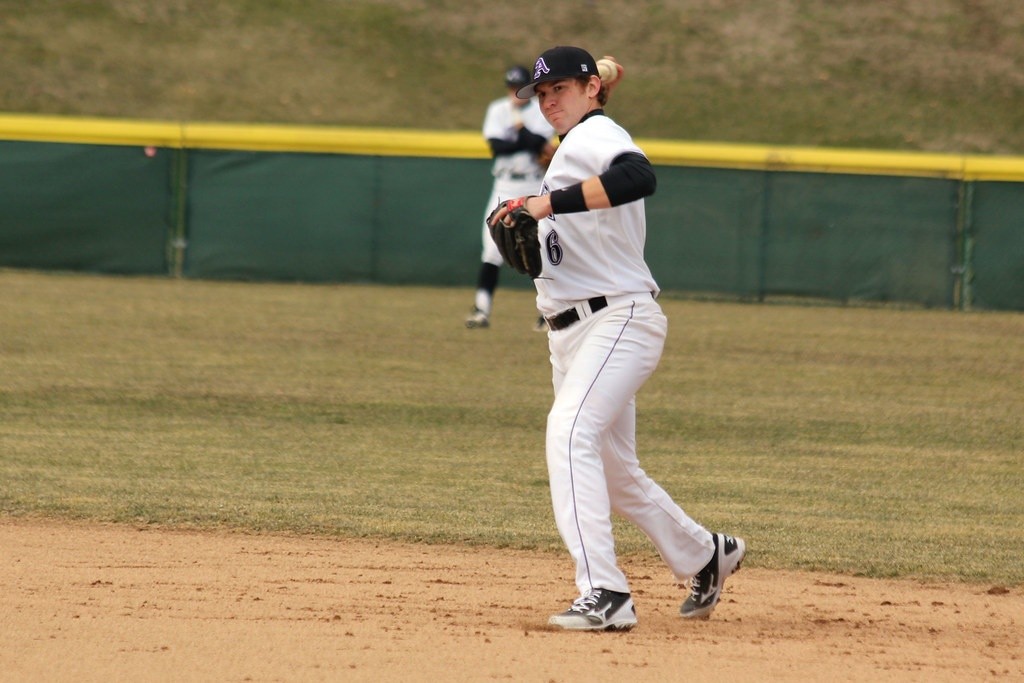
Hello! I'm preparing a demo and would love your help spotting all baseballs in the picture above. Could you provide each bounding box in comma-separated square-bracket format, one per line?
[594, 58, 619, 85]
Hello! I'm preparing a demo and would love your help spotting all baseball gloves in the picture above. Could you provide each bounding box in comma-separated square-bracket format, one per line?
[484, 194, 546, 280]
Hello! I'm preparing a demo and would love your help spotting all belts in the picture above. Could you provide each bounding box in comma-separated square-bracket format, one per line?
[510, 172, 540, 180]
[545, 296, 608, 331]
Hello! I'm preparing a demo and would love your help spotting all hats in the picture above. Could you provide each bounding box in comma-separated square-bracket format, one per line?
[505, 66, 531, 86]
[515, 45, 600, 99]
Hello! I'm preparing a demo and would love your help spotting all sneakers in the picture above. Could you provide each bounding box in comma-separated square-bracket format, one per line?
[547, 588, 638, 632]
[677, 532, 747, 618]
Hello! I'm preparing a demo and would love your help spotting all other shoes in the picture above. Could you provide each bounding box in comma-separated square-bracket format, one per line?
[465, 304, 489, 328]
[532, 309, 550, 332]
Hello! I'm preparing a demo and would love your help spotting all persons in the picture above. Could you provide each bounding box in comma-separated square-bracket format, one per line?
[464, 64, 554, 333]
[490, 45, 746, 633]
